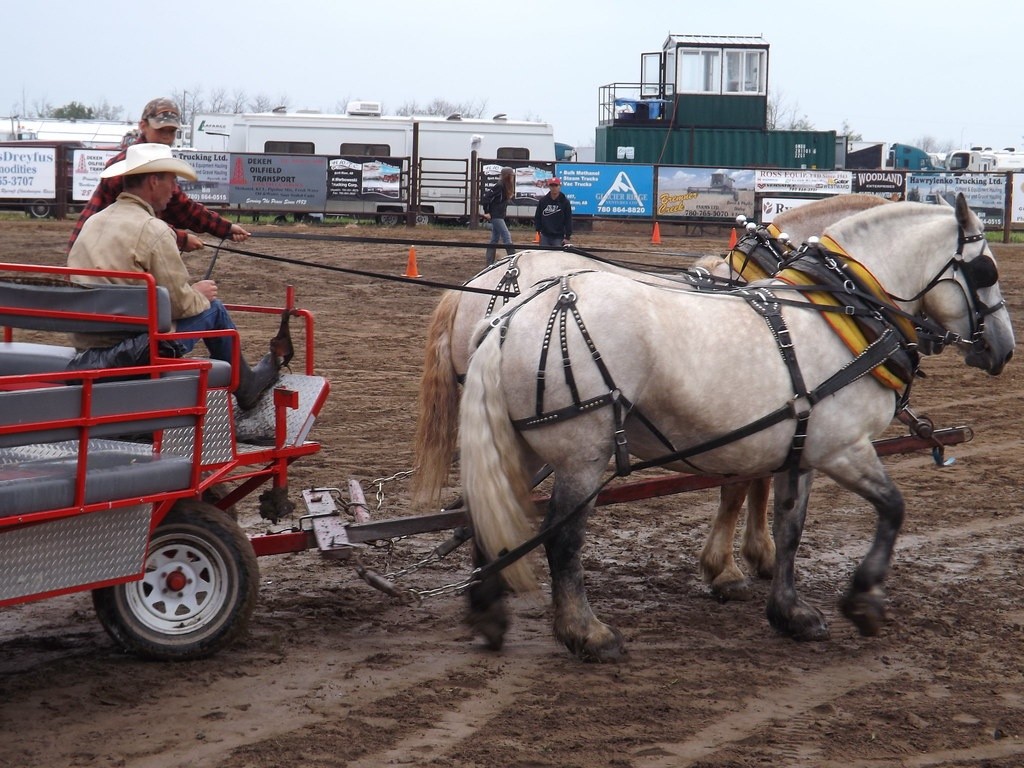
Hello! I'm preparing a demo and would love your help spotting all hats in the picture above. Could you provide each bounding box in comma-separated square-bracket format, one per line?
[100, 143, 197, 181]
[548, 177, 560, 185]
[141, 97, 184, 131]
[501, 167, 515, 176]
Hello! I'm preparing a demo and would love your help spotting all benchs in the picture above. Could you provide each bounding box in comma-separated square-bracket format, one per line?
[0, 357, 212, 528]
[0, 262, 241, 391]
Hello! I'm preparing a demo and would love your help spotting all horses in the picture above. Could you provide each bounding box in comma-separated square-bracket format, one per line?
[412, 184, 902, 603]
[455, 190, 1017, 661]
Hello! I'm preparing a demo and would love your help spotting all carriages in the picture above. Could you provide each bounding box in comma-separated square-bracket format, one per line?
[0, 192, 1016, 666]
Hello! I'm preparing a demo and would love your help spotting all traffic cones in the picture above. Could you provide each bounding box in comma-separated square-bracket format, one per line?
[650, 221, 662, 244]
[725, 227, 737, 251]
[401, 245, 422, 278]
[532, 231, 541, 243]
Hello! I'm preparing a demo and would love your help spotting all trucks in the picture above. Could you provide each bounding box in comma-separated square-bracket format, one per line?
[944, 147, 1024, 177]
[0, 116, 192, 219]
[190, 102, 578, 227]
[847, 141, 935, 176]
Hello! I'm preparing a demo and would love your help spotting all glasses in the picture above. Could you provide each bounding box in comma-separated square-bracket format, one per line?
[549, 183, 559, 187]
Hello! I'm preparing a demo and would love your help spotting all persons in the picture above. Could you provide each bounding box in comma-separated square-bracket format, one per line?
[66, 98, 253, 263]
[483, 166, 517, 268]
[67, 141, 281, 411]
[535, 177, 573, 247]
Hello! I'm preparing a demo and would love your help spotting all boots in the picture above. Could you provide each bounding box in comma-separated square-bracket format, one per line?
[208, 336, 280, 410]
[485, 248, 496, 266]
[506, 248, 515, 255]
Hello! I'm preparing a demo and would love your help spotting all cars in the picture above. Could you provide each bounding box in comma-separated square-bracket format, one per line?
[920, 194, 938, 203]
